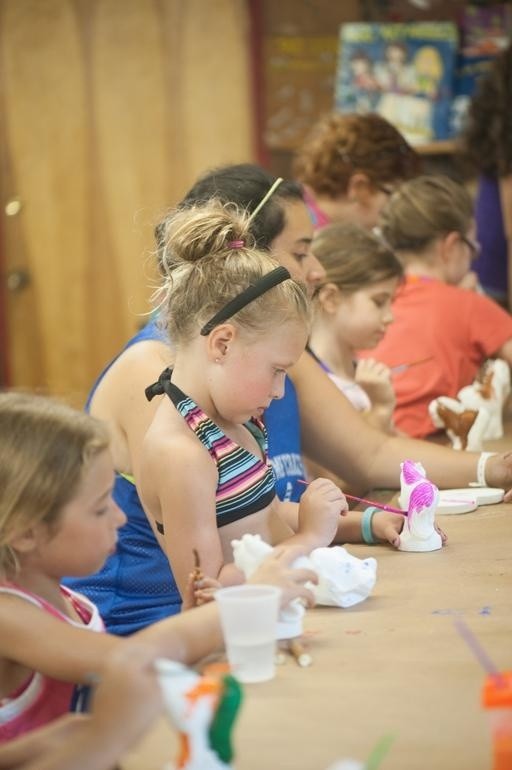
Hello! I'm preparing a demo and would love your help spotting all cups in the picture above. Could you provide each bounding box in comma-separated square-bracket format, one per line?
[217, 584, 280, 684]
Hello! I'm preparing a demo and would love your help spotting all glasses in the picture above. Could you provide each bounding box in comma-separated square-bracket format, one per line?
[463, 231, 484, 261]
[375, 182, 393, 197]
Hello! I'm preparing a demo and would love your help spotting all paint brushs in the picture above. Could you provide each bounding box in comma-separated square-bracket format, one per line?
[297, 478, 408, 516]
[193, 549, 202, 605]
[390, 355, 436, 372]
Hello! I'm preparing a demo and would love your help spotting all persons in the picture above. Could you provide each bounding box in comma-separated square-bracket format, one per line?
[0, 390, 320, 769]
[336, 22, 456, 140]
[56, 165, 512, 564]
[140, 203, 449, 597]
[358, 174, 512, 437]
[294, 113, 437, 223]
[297, 217, 438, 439]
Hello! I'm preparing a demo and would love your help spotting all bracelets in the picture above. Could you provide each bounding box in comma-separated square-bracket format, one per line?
[469, 452, 497, 488]
[361, 506, 378, 543]
